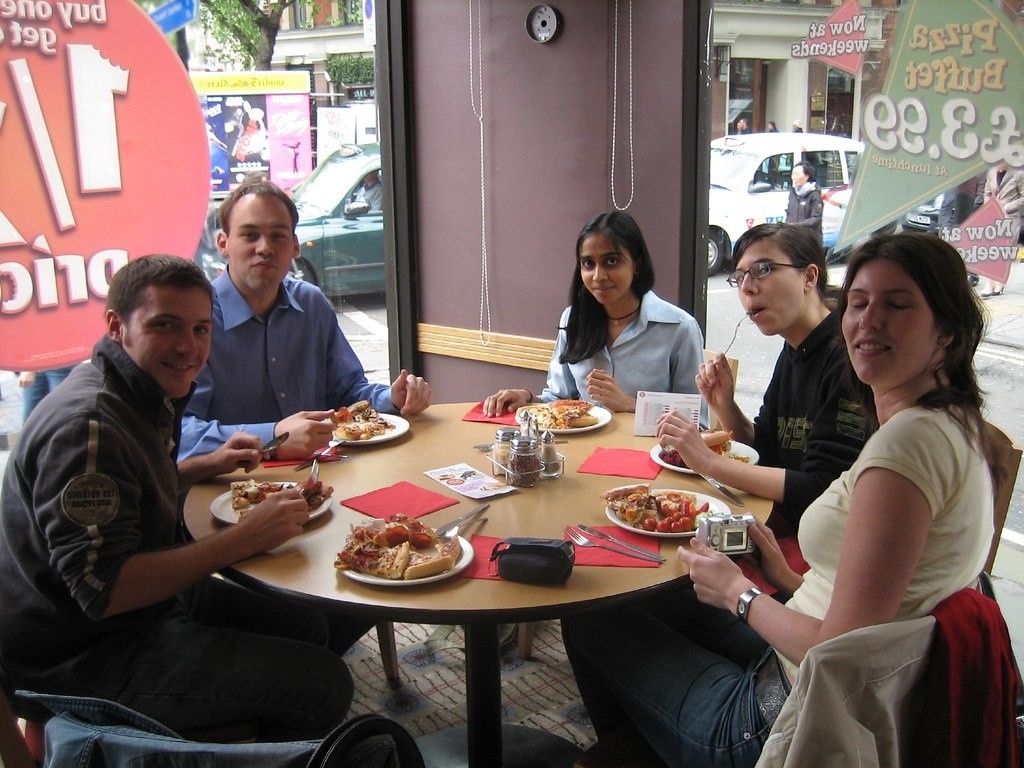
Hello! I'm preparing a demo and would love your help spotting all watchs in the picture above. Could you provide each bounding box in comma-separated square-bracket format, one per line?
[736, 587, 764, 624]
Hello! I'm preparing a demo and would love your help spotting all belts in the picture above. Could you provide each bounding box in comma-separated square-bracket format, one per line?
[753, 652, 789, 725]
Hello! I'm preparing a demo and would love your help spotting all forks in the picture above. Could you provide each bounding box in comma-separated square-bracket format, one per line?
[292, 440, 347, 472]
[563, 524, 663, 562]
[723, 313, 750, 355]
[299, 458, 320, 495]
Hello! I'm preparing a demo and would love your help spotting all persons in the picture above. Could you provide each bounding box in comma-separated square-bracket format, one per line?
[768, 121, 779, 133]
[937, 158, 1024, 300]
[655, 221, 881, 542]
[1, 251, 358, 768]
[736, 119, 752, 135]
[173, 180, 434, 460]
[791, 120, 803, 133]
[785, 160, 824, 248]
[561, 231, 999, 768]
[482, 210, 710, 431]
[354, 170, 383, 211]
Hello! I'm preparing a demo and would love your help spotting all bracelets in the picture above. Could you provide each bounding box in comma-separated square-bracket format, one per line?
[522, 388, 534, 403]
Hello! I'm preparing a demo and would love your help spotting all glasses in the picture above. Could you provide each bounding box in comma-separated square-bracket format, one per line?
[726, 262, 802, 288]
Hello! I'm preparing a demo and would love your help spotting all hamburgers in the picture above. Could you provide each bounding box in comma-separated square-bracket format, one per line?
[699, 428, 732, 455]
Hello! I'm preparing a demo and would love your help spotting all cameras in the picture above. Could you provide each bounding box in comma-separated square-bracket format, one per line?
[694, 513, 756, 554]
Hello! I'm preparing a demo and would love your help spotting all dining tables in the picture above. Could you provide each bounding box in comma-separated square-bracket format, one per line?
[183, 402, 776, 767]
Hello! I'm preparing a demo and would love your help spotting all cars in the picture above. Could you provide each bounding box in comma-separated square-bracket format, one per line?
[210, 143, 386, 300]
[708, 132, 868, 277]
[901, 192, 949, 235]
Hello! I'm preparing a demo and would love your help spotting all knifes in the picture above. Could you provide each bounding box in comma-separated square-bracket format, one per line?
[700, 474, 745, 507]
[236, 432, 290, 465]
[576, 525, 666, 561]
[434, 504, 489, 536]
[313, 451, 348, 458]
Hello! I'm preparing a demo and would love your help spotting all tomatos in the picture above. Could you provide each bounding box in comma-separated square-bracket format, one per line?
[644, 512, 693, 533]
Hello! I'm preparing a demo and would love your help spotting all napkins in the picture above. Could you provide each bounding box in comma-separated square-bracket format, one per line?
[340, 482, 459, 522]
[259, 436, 341, 466]
[462, 396, 520, 426]
[579, 448, 662, 481]
[460, 530, 512, 582]
[565, 521, 665, 568]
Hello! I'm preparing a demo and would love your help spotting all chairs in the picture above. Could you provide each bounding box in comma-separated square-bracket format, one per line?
[743, 578, 985, 766]
[43, 695, 426, 767]
[966, 415, 1023, 576]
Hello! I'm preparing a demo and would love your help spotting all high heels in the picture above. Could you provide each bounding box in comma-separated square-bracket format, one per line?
[981, 287, 1004, 297]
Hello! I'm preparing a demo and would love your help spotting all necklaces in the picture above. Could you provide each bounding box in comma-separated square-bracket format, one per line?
[603, 298, 640, 326]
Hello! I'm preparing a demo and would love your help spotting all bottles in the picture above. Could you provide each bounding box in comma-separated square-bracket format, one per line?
[493, 409, 560, 486]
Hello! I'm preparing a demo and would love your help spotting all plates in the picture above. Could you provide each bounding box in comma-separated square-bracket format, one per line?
[210, 481, 332, 525]
[649, 433, 760, 474]
[319, 412, 411, 447]
[514, 405, 612, 435]
[606, 488, 731, 537]
[337, 528, 476, 586]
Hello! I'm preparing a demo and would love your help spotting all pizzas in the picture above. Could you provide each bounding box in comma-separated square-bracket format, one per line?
[231, 478, 333, 521]
[601, 484, 696, 526]
[516, 400, 598, 429]
[333, 513, 460, 580]
[331, 400, 396, 440]
